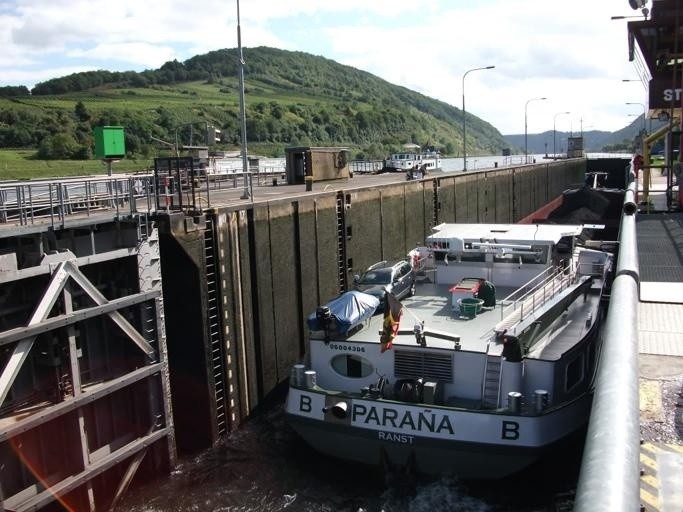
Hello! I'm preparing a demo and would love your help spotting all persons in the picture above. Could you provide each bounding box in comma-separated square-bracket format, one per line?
[419, 163, 427, 178]
[430, 242, 439, 249]
[406, 167, 415, 181]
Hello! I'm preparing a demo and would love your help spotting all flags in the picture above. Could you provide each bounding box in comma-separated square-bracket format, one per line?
[378, 292, 405, 354]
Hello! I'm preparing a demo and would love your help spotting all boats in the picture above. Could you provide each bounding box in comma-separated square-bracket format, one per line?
[385, 142, 441, 172]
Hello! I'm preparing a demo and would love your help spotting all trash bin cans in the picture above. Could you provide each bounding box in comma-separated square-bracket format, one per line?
[249, 156, 259, 172]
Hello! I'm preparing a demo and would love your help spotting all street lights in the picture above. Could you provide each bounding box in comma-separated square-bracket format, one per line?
[524, 97, 546, 164]
[553, 111, 570, 161]
[622, 79, 652, 136]
[462, 66, 495, 172]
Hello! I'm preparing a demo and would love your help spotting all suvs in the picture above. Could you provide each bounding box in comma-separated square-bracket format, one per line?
[353, 260, 417, 311]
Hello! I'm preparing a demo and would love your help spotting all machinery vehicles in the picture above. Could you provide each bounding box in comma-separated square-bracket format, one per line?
[282, 171, 626, 487]
